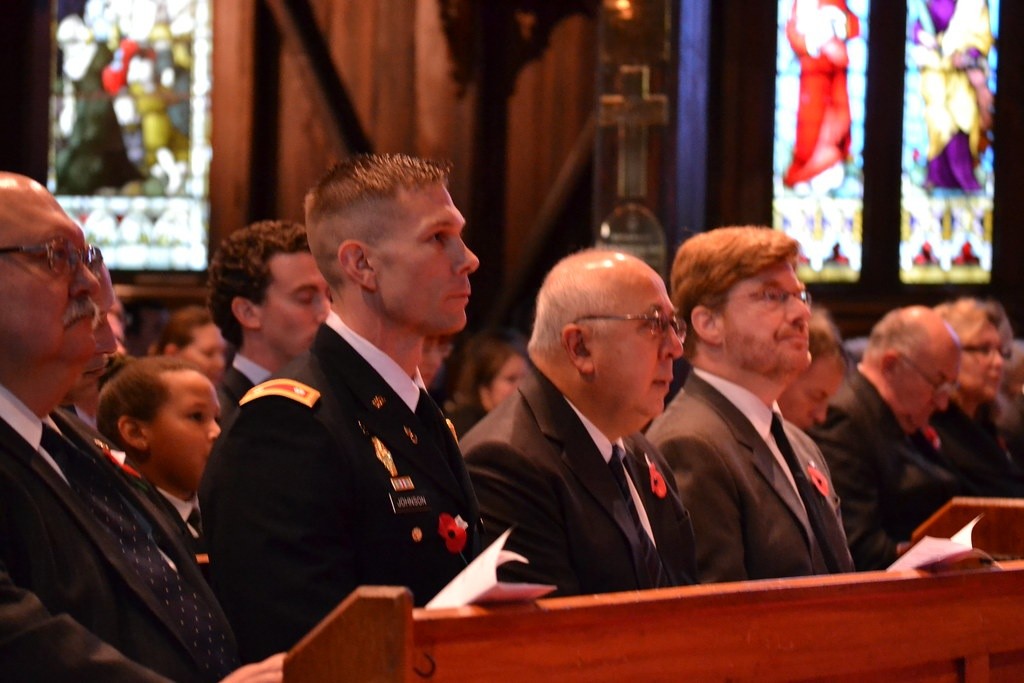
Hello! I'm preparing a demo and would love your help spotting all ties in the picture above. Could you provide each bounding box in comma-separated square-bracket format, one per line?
[38, 420, 240, 682]
[609, 445, 670, 590]
[416, 385, 484, 564]
[770, 411, 847, 574]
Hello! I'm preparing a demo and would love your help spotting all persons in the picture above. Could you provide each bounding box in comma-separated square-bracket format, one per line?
[0, 154, 1024, 683]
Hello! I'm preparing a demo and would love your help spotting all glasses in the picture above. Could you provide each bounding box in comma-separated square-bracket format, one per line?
[898, 351, 961, 397]
[706, 279, 812, 315]
[0, 235, 104, 283]
[960, 340, 1012, 359]
[559, 305, 687, 345]
